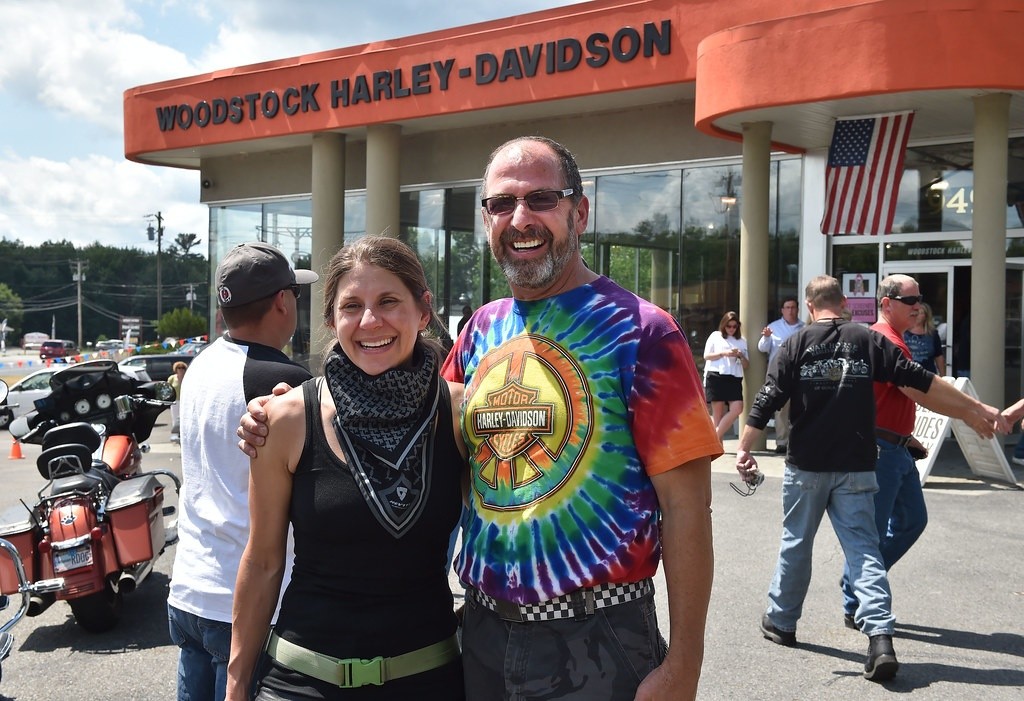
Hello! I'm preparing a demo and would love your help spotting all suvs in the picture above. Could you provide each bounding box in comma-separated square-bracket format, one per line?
[23, 331, 50, 353]
[0, 368, 101, 431]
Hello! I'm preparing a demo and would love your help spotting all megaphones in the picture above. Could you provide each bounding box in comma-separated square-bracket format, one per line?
[202, 179, 211, 189]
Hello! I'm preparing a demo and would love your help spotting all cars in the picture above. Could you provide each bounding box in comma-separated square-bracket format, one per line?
[96, 339, 123, 352]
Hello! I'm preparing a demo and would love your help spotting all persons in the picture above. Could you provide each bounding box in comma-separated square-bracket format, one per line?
[236, 135, 724, 700]
[458, 305, 473, 337]
[167, 243, 320, 701]
[902, 303, 946, 376]
[221, 236, 467, 700]
[995, 399, 1024, 466]
[839, 274, 996, 631]
[757, 297, 807, 453]
[734, 274, 1008, 682]
[167, 361, 188, 442]
[701, 311, 750, 441]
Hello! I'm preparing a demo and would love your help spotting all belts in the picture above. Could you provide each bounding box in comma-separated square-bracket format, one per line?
[875, 428, 913, 449]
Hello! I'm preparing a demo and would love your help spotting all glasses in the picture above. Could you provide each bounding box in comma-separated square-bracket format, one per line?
[726, 325, 737, 329]
[879, 294, 923, 306]
[481, 189, 574, 215]
[291, 284, 303, 299]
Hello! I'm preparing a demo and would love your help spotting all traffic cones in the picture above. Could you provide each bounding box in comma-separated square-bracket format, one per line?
[11, 440, 22, 459]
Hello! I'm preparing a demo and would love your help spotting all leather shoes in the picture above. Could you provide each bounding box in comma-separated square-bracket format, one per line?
[759, 611, 797, 646]
[863, 635, 900, 681]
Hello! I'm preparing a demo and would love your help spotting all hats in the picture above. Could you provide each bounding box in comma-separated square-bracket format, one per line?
[215, 243, 319, 307]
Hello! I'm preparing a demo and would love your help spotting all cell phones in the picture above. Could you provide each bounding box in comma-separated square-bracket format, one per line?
[732, 349, 738, 353]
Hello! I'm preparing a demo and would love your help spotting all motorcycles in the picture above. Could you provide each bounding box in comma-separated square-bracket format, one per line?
[0, 361, 185, 631]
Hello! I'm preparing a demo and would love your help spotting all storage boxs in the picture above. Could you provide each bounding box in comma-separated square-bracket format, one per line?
[0, 502, 38, 595]
[104, 474, 165, 566]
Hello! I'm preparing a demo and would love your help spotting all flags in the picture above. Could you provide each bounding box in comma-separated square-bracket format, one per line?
[819, 108, 916, 237]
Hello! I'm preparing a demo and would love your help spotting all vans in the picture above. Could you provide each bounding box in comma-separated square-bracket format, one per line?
[113, 355, 198, 385]
[39, 338, 75, 360]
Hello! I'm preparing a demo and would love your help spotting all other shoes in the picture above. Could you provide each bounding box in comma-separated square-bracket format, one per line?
[775, 445, 787, 453]
[844, 613, 861, 631]
